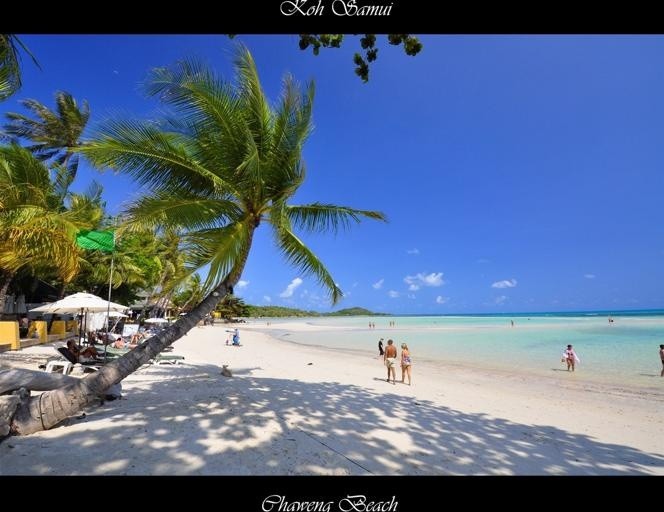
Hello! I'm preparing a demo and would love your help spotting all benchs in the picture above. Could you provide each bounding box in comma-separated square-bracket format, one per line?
[76, 227, 115, 251]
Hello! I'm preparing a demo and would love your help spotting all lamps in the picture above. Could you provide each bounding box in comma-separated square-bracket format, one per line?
[94, 342, 185, 366]
[55, 345, 104, 372]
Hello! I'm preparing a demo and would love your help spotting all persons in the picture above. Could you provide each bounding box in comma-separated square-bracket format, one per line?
[400, 343, 412, 385]
[378, 339, 385, 355]
[227, 328, 239, 346]
[384, 340, 397, 385]
[19, 314, 30, 338]
[611, 317, 613, 323]
[561, 345, 576, 371]
[660, 344, 664, 377]
[67, 329, 152, 363]
[608, 315, 611, 323]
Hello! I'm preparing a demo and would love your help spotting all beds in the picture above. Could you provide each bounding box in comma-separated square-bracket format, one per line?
[28, 291, 130, 361]
[79, 311, 129, 336]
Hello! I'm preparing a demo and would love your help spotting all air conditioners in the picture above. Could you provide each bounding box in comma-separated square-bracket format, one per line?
[221, 364, 232, 378]
